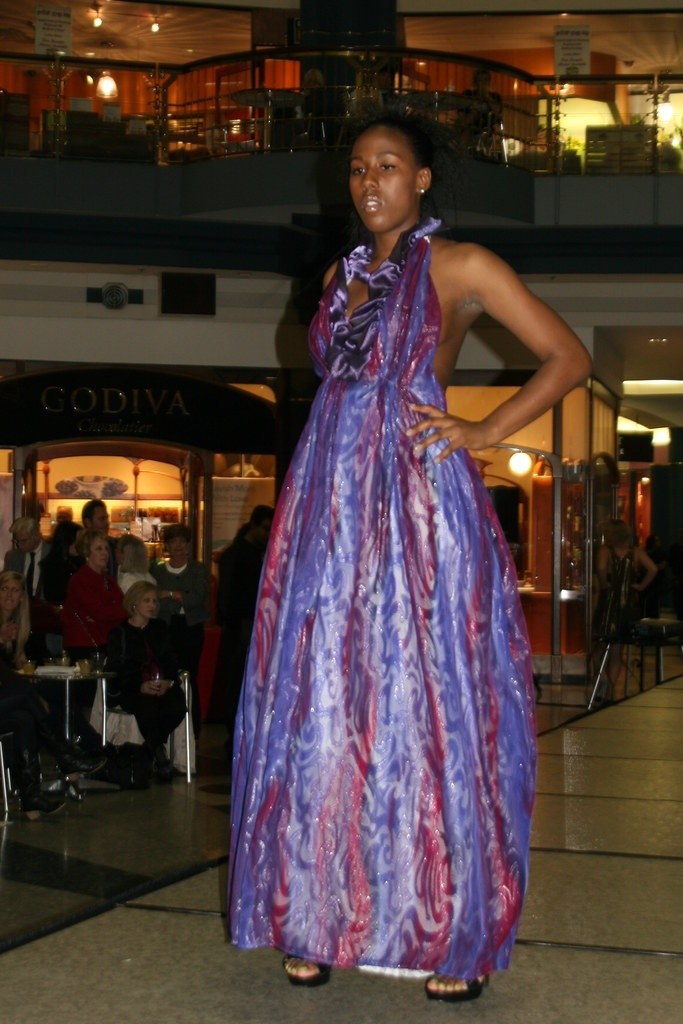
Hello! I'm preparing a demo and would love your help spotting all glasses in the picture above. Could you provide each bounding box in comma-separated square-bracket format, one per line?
[11, 538, 31, 543]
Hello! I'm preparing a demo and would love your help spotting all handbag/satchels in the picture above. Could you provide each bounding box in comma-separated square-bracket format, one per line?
[91, 649, 108, 671]
[106, 626, 127, 709]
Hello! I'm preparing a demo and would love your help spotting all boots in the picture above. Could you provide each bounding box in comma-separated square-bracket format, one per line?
[14, 747, 67, 820]
[36, 713, 109, 782]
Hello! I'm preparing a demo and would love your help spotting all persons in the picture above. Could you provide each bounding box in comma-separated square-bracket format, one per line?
[642, 527, 683, 620]
[590, 518, 658, 704]
[300, 69, 324, 139]
[227, 113, 594, 1004]
[453, 69, 502, 151]
[1, 500, 276, 815]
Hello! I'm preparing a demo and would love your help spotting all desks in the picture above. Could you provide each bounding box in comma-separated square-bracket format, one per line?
[405, 89, 473, 122]
[232, 89, 305, 154]
[12, 669, 118, 747]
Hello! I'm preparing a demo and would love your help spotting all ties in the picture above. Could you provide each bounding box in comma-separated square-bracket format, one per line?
[26, 552, 36, 599]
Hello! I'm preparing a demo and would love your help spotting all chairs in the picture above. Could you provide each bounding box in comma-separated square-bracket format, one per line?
[88, 652, 198, 783]
[444, 110, 503, 163]
[595, 554, 683, 698]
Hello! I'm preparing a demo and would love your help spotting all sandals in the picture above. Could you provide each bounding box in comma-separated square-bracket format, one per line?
[282, 954, 331, 987]
[424, 974, 489, 1003]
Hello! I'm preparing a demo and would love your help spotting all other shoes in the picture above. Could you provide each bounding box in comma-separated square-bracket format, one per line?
[130, 753, 150, 790]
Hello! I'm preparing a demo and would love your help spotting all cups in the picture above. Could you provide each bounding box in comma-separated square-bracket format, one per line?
[92, 652, 105, 672]
[151, 672, 164, 680]
[43, 657, 56, 666]
[21, 658, 35, 675]
[56, 657, 70, 666]
[79, 659, 91, 676]
